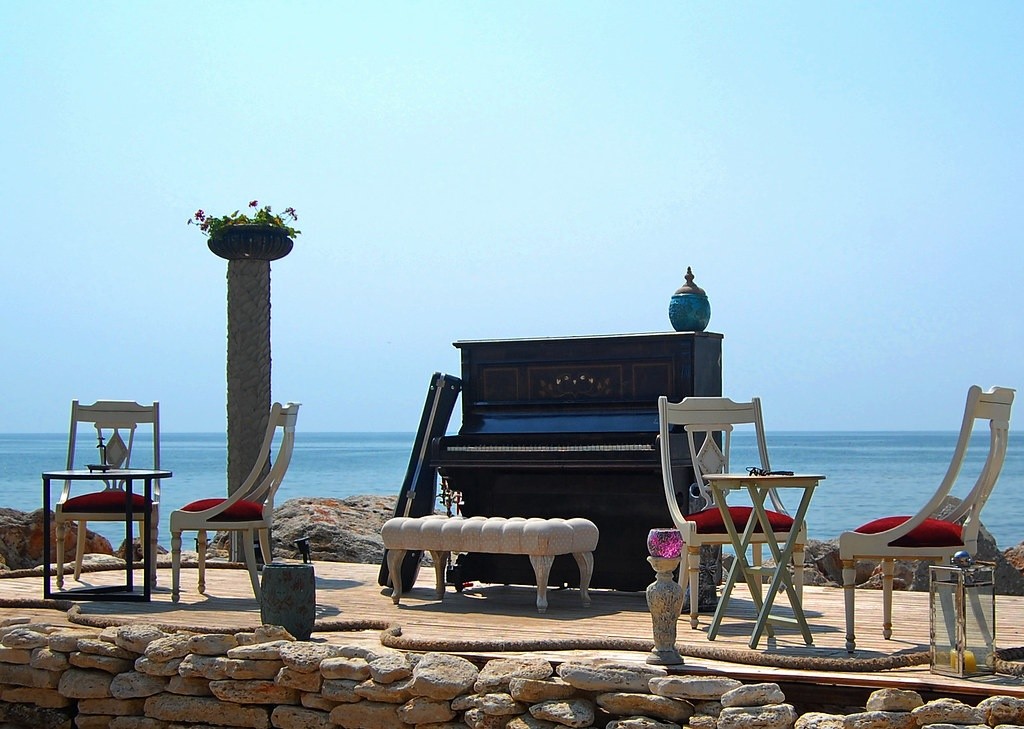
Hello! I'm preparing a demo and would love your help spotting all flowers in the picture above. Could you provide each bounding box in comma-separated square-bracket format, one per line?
[189, 199, 301, 241]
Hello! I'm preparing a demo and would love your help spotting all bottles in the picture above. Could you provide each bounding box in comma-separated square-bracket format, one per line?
[260, 563, 316, 641]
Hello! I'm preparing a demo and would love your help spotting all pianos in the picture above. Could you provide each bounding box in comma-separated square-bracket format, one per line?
[428, 330, 725, 593]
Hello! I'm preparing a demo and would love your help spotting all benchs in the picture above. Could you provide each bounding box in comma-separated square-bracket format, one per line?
[379, 516, 599, 612]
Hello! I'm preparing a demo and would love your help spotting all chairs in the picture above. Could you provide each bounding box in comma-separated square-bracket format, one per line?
[840, 386, 1014, 657]
[170, 401, 300, 606]
[56, 399, 161, 588]
[657, 397, 810, 632]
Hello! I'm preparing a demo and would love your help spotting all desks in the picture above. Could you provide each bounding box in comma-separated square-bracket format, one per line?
[701, 472, 827, 648]
[40, 471, 171, 603]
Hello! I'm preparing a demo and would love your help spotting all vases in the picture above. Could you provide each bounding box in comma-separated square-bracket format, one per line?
[259, 565, 314, 641]
[646, 556, 684, 666]
[207, 226, 293, 260]
